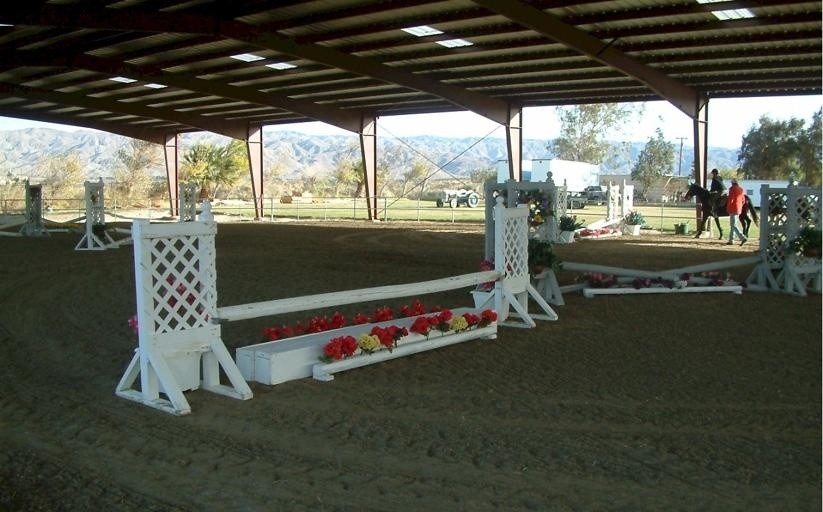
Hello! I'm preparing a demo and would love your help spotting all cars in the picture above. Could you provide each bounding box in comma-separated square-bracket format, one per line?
[582, 186, 609, 199]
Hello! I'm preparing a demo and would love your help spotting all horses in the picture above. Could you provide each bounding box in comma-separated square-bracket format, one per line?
[683, 179, 760, 240]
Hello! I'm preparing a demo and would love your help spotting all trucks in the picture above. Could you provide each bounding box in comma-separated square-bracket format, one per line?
[531, 159, 599, 208]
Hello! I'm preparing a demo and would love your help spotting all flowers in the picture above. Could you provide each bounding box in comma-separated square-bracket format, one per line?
[519, 192, 556, 226]
[575, 271, 734, 289]
[261, 299, 497, 364]
[126, 268, 209, 336]
[481, 257, 511, 291]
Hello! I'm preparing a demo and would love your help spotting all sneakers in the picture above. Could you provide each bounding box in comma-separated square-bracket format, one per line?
[740, 239, 746, 246]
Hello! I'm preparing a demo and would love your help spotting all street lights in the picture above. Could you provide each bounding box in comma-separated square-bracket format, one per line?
[674, 137, 689, 176]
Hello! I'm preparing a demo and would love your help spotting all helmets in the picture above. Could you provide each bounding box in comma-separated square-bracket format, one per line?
[712, 169, 719, 174]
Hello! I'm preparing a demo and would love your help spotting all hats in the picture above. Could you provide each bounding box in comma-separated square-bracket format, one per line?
[732, 179, 739, 183]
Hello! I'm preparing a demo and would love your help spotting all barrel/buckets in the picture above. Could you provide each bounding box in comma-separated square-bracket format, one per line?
[673, 222, 690, 235]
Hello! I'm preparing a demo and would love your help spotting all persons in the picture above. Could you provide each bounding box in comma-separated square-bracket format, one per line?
[710, 169, 728, 219]
[727, 177, 747, 246]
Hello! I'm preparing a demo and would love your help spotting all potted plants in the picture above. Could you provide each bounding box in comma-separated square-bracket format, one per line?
[783, 223, 822, 258]
[560, 214, 587, 244]
[624, 209, 646, 236]
[528, 238, 562, 281]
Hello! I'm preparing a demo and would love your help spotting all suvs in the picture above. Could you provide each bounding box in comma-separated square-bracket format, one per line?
[436, 187, 480, 208]
